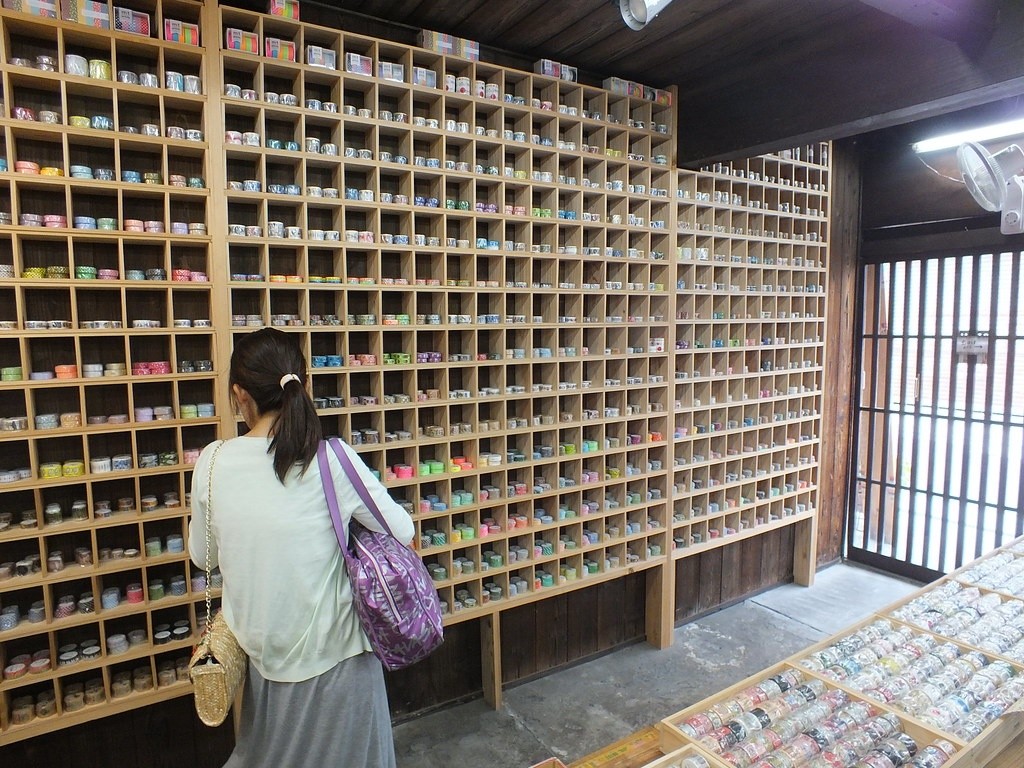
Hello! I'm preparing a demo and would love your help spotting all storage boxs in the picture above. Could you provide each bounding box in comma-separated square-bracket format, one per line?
[60, 0, 110, 30]
[628, 81, 643, 99]
[112, 6, 150, 38]
[656, 89, 671, 105]
[643, 85, 657, 102]
[602, 77, 628, 94]
[3, 0, 57, 19]
[305, 45, 336, 71]
[224, 28, 259, 55]
[265, 37, 295, 62]
[416, 30, 480, 61]
[535, 59, 560, 79]
[164, 18, 199, 47]
[267, 0, 299, 20]
[344, 52, 373, 76]
[413, 66, 436, 89]
[378, 61, 404, 82]
[560, 64, 578, 83]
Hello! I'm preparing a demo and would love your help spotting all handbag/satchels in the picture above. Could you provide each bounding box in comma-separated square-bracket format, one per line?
[317, 436, 445, 672]
[188, 609, 248, 727]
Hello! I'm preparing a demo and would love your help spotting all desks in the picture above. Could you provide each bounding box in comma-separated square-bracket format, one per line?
[566, 723, 1024, 768]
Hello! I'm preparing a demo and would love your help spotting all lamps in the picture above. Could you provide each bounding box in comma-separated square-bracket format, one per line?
[620, 0, 672, 31]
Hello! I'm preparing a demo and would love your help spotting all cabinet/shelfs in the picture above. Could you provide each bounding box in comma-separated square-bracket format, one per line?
[0, 0, 833, 748]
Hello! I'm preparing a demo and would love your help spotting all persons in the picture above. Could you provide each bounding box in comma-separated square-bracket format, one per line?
[187, 327, 416, 768]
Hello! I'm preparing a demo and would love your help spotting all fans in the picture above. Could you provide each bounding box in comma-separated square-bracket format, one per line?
[956, 141, 1024, 235]
